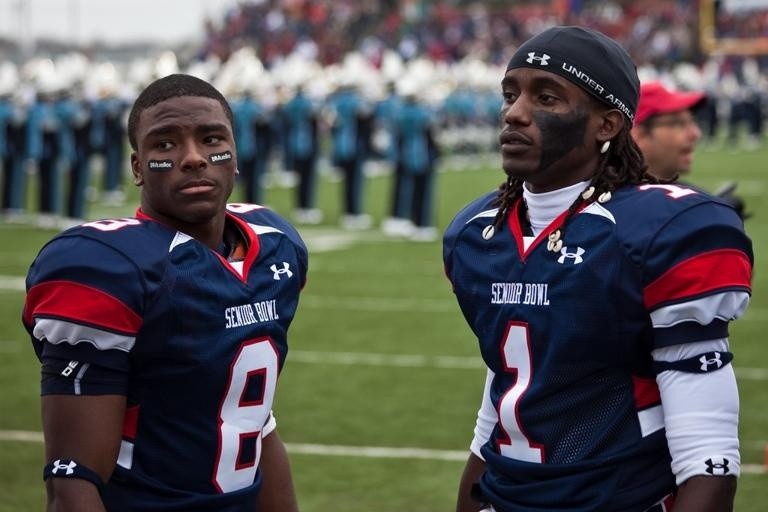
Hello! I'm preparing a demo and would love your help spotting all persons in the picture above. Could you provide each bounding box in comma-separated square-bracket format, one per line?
[0, 80, 131, 230]
[200, 0, 768, 242]
[22, 75, 308, 512]
[443, 26, 754, 512]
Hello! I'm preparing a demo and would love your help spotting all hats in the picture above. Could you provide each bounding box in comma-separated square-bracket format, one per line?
[631, 80, 705, 128]
[504, 22, 641, 124]
